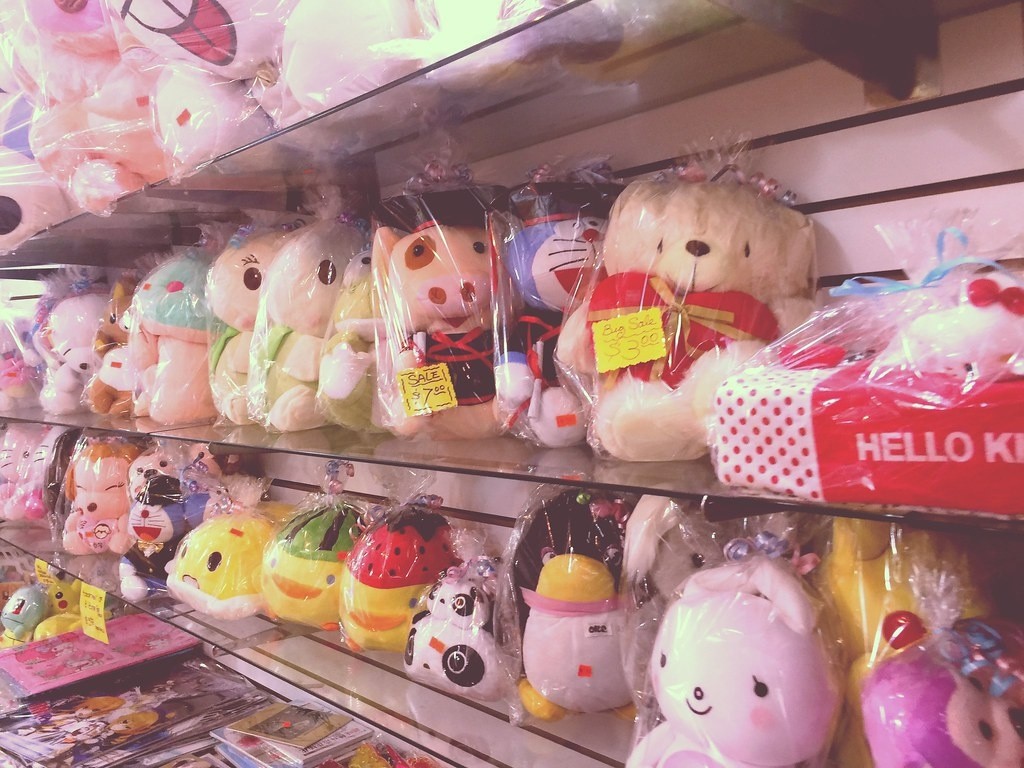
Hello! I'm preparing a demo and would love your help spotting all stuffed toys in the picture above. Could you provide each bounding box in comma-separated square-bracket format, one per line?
[0, 422, 1024, 767]
[0, 579, 112, 650]
[0, 138, 1024, 459]
[0, 0, 622, 256]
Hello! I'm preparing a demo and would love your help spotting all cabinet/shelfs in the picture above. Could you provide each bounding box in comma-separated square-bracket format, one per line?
[0, 0, 1023, 768]
